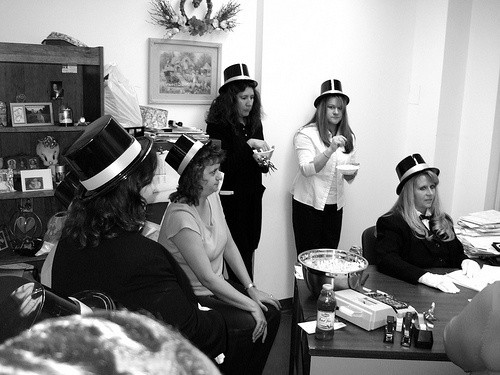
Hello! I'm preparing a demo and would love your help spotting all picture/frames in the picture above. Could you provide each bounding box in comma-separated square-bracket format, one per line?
[0, 168, 16, 194]
[0, 231, 8, 251]
[20, 198, 33, 212]
[10, 102, 54, 127]
[148, 38, 222, 105]
[21, 170, 54, 192]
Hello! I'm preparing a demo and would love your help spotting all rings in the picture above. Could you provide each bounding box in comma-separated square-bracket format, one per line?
[270, 295, 273, 298]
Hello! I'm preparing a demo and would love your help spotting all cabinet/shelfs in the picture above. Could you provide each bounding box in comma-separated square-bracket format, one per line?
[0, 42, 104, 275]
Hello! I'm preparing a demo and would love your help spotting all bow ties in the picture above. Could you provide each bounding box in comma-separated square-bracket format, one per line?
[418, 214, 432, 221]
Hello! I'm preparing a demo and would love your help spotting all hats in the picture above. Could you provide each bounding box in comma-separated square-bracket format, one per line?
[314, 79, 350, 108]
[218, 64, 258, 92]
[0, 275, 114, 346]
[165, 133, 212, 184]
[54, 170, 84, 212]
[64, 115, 154, 202]
[396, 153, 440, 194]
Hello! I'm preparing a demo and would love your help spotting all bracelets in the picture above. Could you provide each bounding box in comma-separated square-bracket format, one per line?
[245, 284, 255, 290]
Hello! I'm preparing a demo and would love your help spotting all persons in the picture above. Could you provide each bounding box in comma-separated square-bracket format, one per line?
[50, 115, 227, 375]
[51, 83, 60, 99]
[444, 280, 500, 375]
[206, 64, 275, 286]
[378, 154, 481, 293]
[158, 135, 282, 375]
[292, 79, 360, 263]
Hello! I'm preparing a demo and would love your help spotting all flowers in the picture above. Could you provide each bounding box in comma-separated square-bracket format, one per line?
[150, 0, 241, 39]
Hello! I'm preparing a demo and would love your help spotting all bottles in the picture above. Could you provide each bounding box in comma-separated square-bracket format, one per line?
[315, 283, 336, 340]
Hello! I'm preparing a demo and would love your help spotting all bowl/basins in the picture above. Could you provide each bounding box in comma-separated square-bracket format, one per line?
[253, 149, 276, 159]
[336, 165, 360, 175]
[298, 248, 369, 296]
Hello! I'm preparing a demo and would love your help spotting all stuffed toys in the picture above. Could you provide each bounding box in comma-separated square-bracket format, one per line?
[36, 135, 59, 175]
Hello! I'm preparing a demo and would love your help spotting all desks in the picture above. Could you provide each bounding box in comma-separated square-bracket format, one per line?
[289, 262, 479, 375]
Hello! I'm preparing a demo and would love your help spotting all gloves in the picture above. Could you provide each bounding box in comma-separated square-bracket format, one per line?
[461, 259, 481, 279]
[418, 272, 460, 293]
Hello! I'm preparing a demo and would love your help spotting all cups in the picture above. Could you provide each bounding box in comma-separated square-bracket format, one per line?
[55, 166, 65, 184]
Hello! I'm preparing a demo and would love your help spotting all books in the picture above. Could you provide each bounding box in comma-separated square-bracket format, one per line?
[145, 126, 209, 142]
